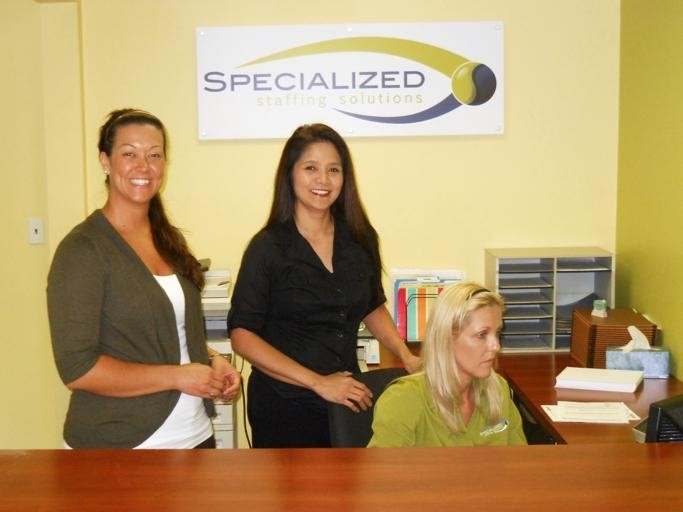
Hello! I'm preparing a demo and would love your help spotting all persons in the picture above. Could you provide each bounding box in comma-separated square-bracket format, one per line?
[366, 283, 530, 446]
[225, 123, 429, 448]
[42, 105, 241, 450]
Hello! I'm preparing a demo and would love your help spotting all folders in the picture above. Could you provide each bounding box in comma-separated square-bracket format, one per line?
[395, 276, 461, 342]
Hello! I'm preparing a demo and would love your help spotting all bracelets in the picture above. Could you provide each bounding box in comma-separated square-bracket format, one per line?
[206, 351, 228, 363]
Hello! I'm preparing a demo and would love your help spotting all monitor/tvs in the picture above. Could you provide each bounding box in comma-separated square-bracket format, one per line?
[646, 394, 683, 442]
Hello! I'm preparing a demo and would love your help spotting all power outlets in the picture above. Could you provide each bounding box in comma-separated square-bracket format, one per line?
[26, 218, 44, 244]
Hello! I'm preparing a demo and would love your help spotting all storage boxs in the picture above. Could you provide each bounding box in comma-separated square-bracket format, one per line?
[571, 306, 655, 369]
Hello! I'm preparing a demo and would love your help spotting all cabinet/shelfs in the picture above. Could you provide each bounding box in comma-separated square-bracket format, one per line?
[484, 247, 612, 354]
[202, 280, 253, 450]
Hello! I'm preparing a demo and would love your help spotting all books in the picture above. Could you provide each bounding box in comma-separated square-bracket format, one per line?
[554, 367, 647, 394]
